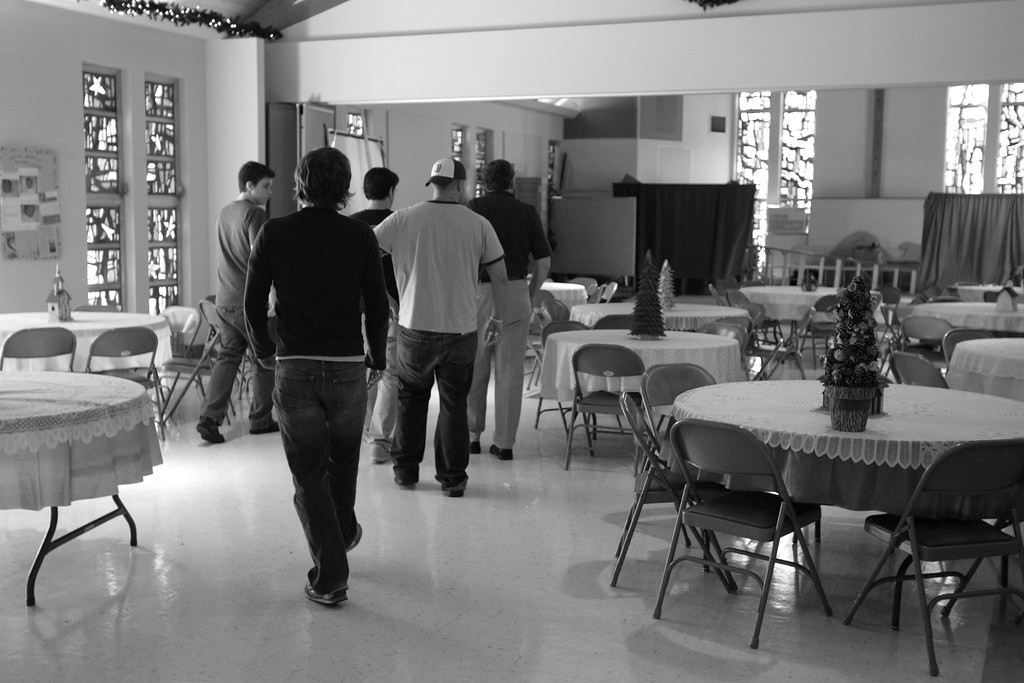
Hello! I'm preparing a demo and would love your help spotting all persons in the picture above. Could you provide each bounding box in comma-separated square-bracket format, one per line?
[195, 161, 280, 443]
[244, 147, 388, 605]
[466, 159, 551, 460]
[347, 159, 510, 497]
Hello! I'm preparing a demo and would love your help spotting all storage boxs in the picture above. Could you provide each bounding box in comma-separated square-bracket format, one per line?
[767, 207, 807, 234]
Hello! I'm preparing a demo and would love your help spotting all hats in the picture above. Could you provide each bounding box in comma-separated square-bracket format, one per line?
[425, 157, 467, 186]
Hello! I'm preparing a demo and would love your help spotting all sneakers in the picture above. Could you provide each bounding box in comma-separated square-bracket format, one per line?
[304, 581, 347, 605]
[347, 524, 362, 553]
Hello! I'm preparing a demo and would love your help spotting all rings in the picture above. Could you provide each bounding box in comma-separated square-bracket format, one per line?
[488, 332, 494, 336]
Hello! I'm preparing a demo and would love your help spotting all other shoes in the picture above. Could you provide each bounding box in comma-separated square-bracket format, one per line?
[249, 420, 279, 434]
[370, 440, 393, 464]
[197, 417, 225, 444]
[488, 444, 515, 461]
[469, 441, 481, 454]
[442, 486, 463, 497]
[395, 476, 416, 489]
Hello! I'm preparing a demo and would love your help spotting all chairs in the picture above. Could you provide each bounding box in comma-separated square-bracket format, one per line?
[160, 297, 253, 429]
[76, 304, 120, 313]
[0, 327, 77, 372]
[85, 326, 173, 441]
[529, 275, 1024, 679]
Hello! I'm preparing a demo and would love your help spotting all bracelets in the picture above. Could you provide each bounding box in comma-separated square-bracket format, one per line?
[490, 316, 503, 324]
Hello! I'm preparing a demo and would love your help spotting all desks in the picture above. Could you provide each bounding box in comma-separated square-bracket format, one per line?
[570, 300, 751, 352]
[538, 282, 588, 311]
[0, 368, 163, 607]
[738, 285, 880, 364]
[945, 338, 1024, 402]
[912, 301, 1024, 334]
[538, 326, 742, 440]
[949, 283, 1024, 301]
[674, 377, 1024, 572]
[0, 310, 172, 374]
